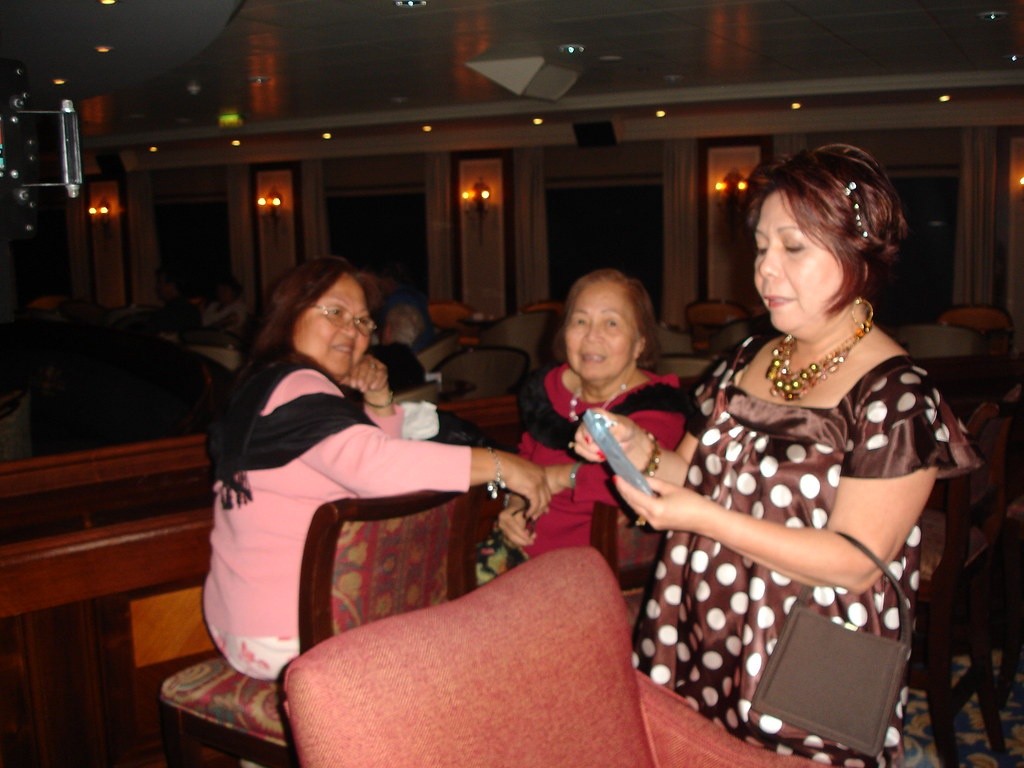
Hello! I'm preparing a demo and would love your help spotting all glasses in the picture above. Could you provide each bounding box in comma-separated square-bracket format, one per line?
[309, 304, 377, 337]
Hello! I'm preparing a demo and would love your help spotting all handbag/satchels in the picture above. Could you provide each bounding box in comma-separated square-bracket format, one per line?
[750, 529, 913, 754]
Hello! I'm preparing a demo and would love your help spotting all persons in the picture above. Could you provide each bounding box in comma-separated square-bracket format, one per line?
[196, 254, 553, 683]
[474, 265, 693, 599]
[569, 143, 943, 768]
[20, 259, 426, 396]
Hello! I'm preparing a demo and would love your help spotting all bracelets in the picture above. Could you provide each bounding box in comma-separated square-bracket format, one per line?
[487, 447, 507, 499]
[570, 460, 584, 489]
[362, 390, 394, 408]
[638, 432, 663, 477]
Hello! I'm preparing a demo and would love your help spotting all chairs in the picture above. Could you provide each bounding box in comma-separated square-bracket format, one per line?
[0, 293, 1024, 768]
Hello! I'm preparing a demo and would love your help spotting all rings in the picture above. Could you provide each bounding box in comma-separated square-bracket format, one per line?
[369, 361, 377, 369]
[569, 441, 576, 449]
[635, 514, 646, 526]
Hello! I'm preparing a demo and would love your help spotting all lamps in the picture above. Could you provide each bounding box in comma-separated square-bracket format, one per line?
[88, 164, 749, 253]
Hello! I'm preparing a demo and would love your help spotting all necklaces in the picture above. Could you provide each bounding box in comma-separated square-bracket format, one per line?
[764, 319, 876, 400]
[568, 377, 632, 424]
